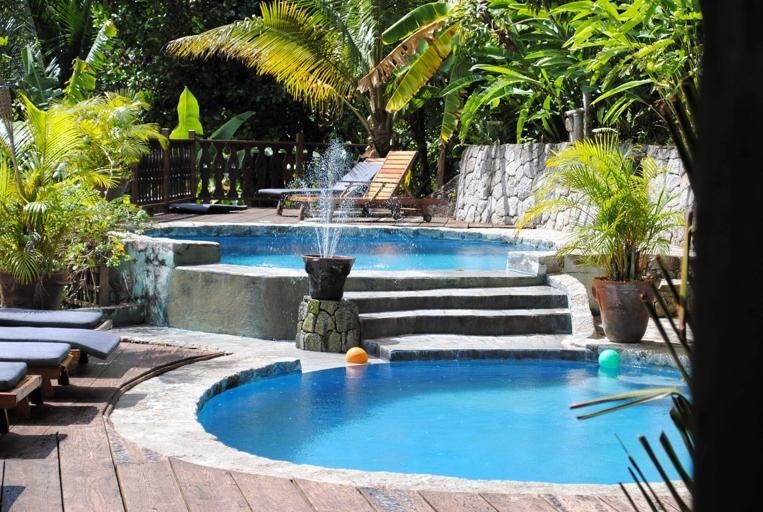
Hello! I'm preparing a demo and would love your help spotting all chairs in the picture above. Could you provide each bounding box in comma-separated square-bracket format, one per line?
[0, 307, 113, 434]
[287, 150, 442, 223]
[257, 157, 386, 215]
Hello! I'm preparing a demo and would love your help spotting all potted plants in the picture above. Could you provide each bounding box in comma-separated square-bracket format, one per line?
[511, 126, 691, 344]
[1, 87, 169, 311]
